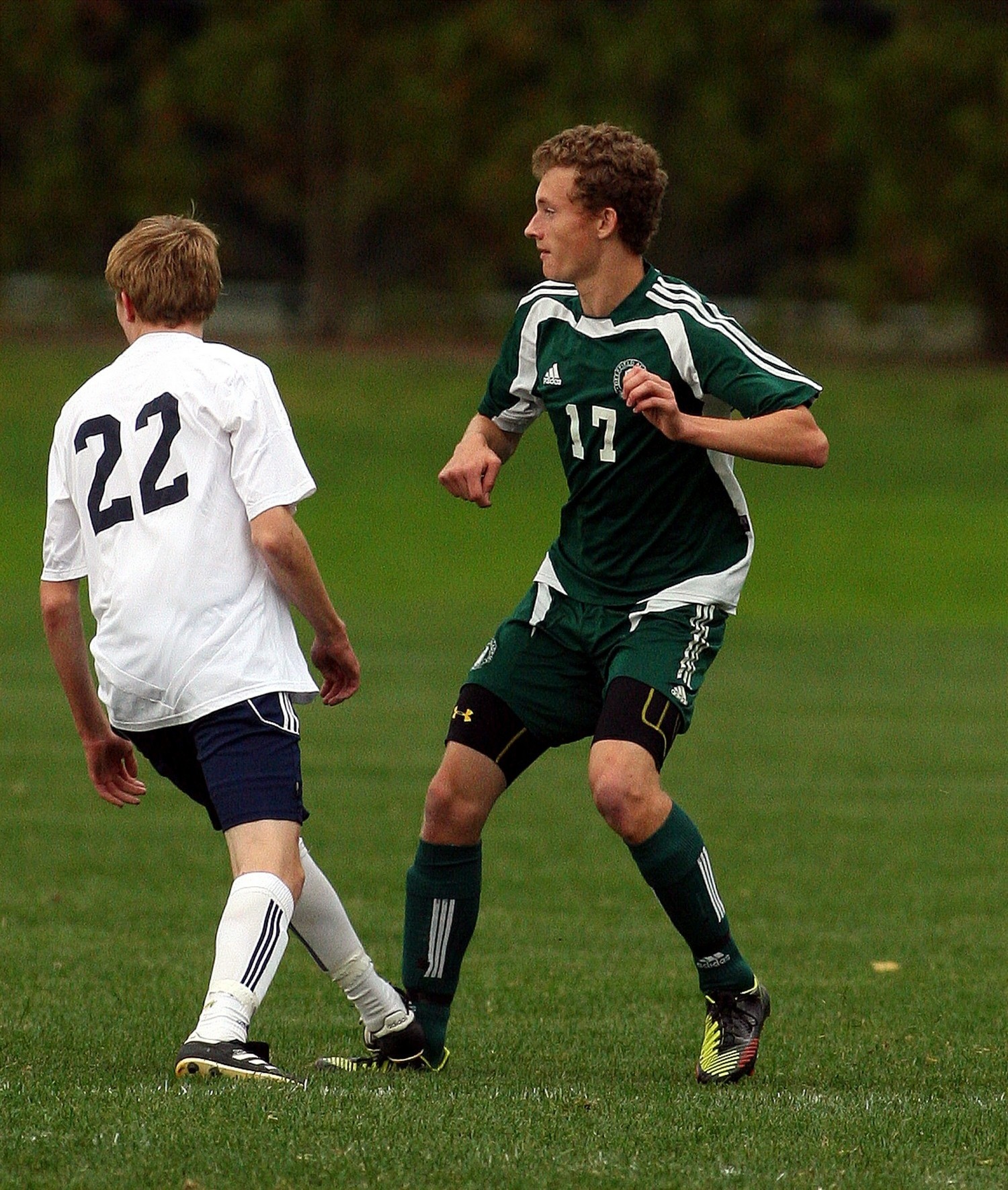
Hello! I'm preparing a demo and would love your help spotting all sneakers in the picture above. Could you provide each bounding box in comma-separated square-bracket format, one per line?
[315, 1046, 450, 1077]
[174, 1040, 304, 1087]
[359, 983, 424, 1061]
[696, 969, 771, 1084]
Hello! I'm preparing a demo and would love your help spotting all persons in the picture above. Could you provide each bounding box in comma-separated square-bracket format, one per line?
[313, 122, 829, 1083]
[39, 216, 423, 1087]
